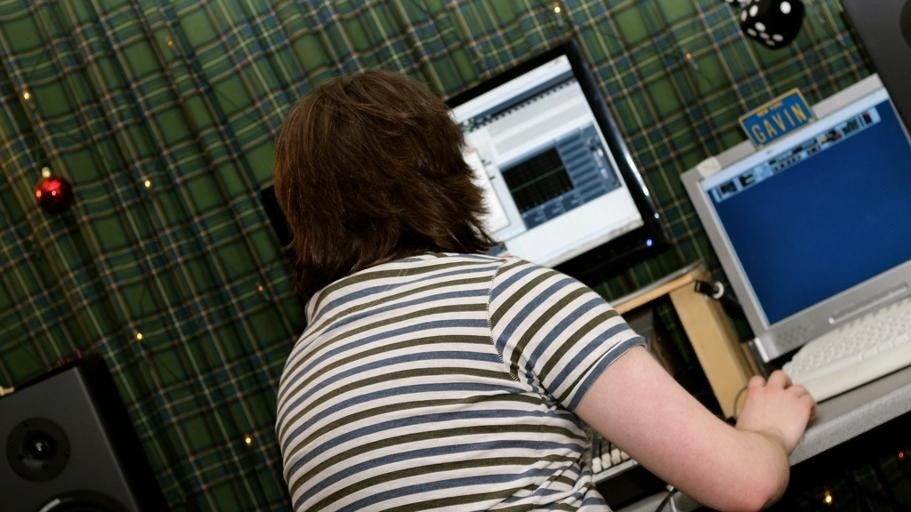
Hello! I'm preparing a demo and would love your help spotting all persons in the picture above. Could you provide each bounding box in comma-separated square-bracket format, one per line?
[273, 69, 820, 512]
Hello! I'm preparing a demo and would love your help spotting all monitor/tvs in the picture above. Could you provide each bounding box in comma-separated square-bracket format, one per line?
[259, 36, 675, 297]
[678, 72, 911, 363]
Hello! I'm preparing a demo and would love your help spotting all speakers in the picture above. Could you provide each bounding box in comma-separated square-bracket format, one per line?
[1, 352, 174, 512]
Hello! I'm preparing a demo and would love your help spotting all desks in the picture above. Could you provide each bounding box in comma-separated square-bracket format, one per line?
[619, 366, 911, 512]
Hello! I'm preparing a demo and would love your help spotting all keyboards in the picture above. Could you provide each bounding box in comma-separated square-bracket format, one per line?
[776, 296, 911, 403]
